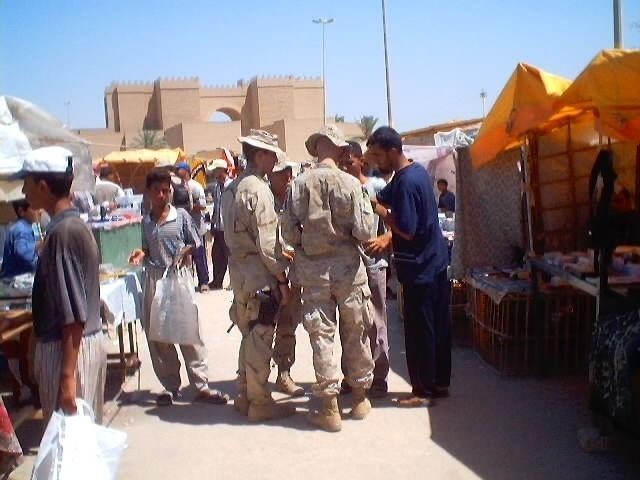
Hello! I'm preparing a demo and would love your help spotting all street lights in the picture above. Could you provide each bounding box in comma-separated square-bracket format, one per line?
[312, 17, 335, 127]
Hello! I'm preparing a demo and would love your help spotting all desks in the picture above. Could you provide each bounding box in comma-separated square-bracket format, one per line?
[526, 246, 640, 396]
[0, 265, 146, 479]
[91, 220, 142, 267]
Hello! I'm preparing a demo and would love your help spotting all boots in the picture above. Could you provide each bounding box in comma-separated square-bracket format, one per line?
[232, 359, 389, 431]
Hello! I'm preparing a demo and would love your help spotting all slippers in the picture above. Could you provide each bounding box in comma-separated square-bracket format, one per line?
[196, 388, 230, 404]
[199, 283, 209, 293]
[158, 390, 176, 406]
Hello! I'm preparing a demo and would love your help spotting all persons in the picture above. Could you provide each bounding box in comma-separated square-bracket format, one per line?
[92, 167, 125, 204]
[7, 145, 108, 434]
[204, 124, 455, 431]
[127, 162, 231, 408]
[0, 199, 46, 282]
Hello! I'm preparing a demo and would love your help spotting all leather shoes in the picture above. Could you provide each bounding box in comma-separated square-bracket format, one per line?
[398, 393, 435, 407]
[436, 385, 449, 396]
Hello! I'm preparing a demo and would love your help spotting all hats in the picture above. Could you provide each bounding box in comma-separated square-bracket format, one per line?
[176, 163, 189, 169]
[7, 146, 75, 182]
[237, 124, 353, 174]
[207, 159, 228, 172]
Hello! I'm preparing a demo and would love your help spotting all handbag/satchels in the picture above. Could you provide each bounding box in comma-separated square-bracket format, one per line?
[148, 262, 204, 346]
[30, 397, 129, 480]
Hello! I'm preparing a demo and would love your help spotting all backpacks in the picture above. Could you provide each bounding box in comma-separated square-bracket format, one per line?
[171, 177, 189, 205]
